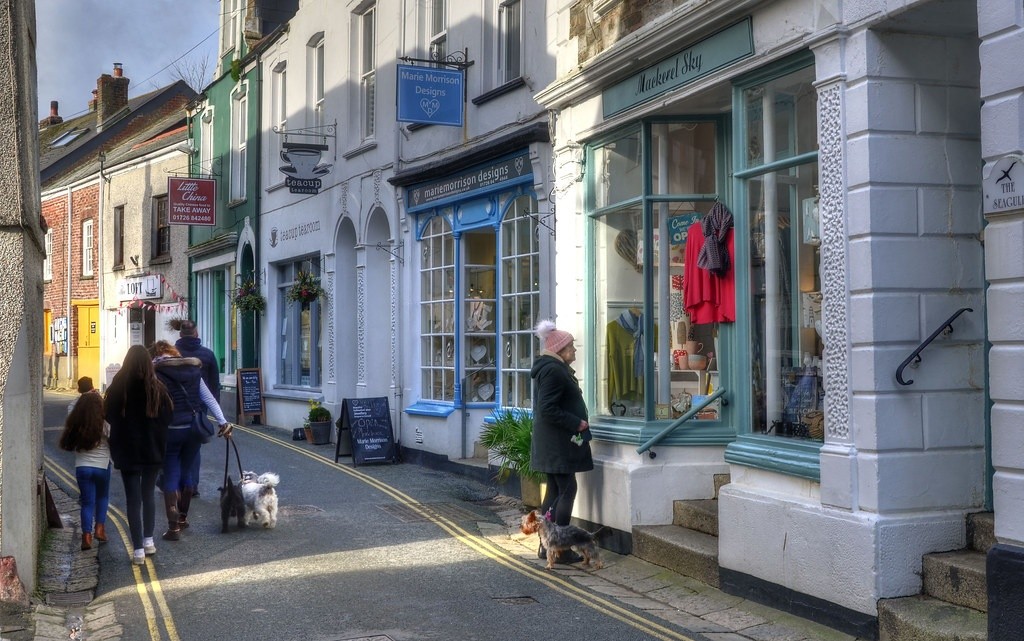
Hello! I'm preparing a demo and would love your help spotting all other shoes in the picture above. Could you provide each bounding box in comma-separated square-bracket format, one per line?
[538, 545, 584, 562]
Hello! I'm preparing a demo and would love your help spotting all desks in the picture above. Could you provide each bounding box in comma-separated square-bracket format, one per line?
[653, 369, 707, 401]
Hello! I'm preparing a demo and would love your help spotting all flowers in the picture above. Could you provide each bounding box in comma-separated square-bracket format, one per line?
[284, 269, 332, 304]
[231, 271, 268, 314]
[299, 399, 321, 427]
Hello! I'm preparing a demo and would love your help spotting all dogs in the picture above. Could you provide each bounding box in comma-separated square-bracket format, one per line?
[238, 470, 280, 529]
[217, 475, 245, 534]
[518, 510, 607, 571]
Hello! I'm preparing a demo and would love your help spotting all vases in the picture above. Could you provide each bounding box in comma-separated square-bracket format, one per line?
[678, 340, 708, 370]
[304, 428, 314, 443]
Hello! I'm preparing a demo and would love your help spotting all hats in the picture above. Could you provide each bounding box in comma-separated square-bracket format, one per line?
[533, 318, 574, 354]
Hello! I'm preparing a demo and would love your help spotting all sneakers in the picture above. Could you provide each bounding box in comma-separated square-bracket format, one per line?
[145, 546, 156, 553]
[133, 553, 145, 564]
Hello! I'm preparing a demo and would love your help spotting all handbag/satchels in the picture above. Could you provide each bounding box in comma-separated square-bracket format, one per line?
[191, 408, 214, 444]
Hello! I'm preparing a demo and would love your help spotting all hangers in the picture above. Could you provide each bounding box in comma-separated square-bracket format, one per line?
[627, 299, 641, 319]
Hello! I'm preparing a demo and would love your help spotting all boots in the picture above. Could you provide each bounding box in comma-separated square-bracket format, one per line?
[81, 533, 92, 550]
[179, 486, 194, 529]
[94, 523, 109, 541]
[163, 489, 181, 539]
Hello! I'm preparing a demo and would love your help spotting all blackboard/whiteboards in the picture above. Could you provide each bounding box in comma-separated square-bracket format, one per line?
[237, 368, 266, 416]
[344, 396, 398, 465]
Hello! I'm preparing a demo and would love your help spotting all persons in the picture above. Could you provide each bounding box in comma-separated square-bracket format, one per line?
[103, 344, 174, 565]
[149, 340, 231, 540]
[174, 321, 221, 498]
[59, 376, 114, 551]
[530, 319, 594, 564]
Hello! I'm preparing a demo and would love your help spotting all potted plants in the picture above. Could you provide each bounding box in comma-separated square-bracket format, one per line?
[308, 407, 332, 444]
[482, 401, 551, 507]
